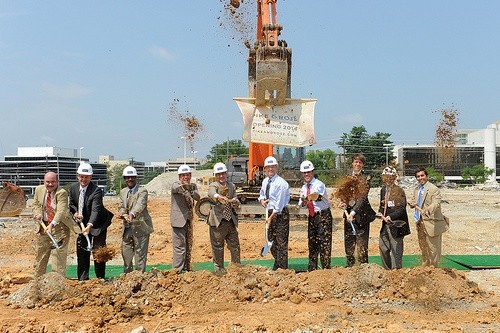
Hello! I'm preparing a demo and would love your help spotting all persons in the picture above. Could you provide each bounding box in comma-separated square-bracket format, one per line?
[33, 172, 73, 278]
[298, 160, 333, 272]
[117, 165, 154, 274]
[377, 166, 411, 270]
[68, 162, 114, 282]
[337, 154, 377, 268]
[258, 156, 291, 271]
[206, 161, 242, 272]
[412, 167, 447, 268]
[170, 165, 200, 271]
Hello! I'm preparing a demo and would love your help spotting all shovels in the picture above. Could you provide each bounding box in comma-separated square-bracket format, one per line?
[259, 199, 273, 257]
[413, 203, 444, 224]
[123, 214, 143, 229]
[339, 207, 364, 236]
[37, 216, 66, 252]
[378, 209, 406, 228]
[73, 212, 95, 252]
[302, 190, 320, 208]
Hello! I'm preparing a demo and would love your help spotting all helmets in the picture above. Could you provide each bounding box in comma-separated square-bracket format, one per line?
[177, 165, 191, 174]
[76, 163, 93, 175]
[300, 160, 314, 173]
[213, 162, 228, 174]
[382, 166, 398, 176]
[123, 166, 138, 176]
[264, 156, 279, 167]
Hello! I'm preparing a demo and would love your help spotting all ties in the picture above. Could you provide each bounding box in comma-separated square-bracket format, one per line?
[127, 190, 133, 208]
[349, 174, 358, 206]
[384, 190, 388, 216]
[46, 192, 55, 225]
[79, 190, 85, 215]
[266, 180, 272, 204]
[414, 187, 425, 221]
[225, 192, 231, 221]
[307, 184, 316, 217]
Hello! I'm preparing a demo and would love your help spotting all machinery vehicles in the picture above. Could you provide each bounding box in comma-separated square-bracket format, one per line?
[194, 0, 319, 223]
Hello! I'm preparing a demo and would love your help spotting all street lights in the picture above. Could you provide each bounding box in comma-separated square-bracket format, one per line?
[80, 147, 85, 164]
[383, 144, 391, 167]
[180, 136, 186, 165]
[191, 151, 198, 170]
